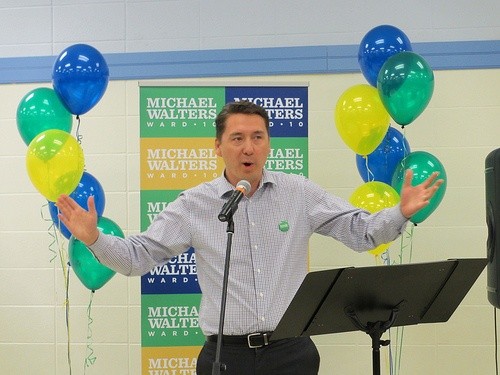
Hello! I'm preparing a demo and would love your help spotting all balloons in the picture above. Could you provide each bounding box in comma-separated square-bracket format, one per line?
[357, 24, 414, 97]
[355, 126, 411, 195]
[376, 53, 435, 127]
[333, 85, 392, 158]
[391, 152, 448, 227]
[51, 43, 109, 119]
[25, 129, 85, 204]
[16, 87, 74, 162]
[48, 171, 106, 242]
[67, 216, 126, 293]
[346, 182, 407, 256]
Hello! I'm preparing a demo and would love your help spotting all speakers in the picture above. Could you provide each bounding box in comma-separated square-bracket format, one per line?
[485, 148, 500, 308]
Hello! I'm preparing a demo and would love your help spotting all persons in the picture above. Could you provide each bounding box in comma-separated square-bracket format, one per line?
[56, 99, 445, 375]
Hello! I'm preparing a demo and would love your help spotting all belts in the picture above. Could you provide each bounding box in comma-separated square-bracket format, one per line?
[208, 333, 270, 349]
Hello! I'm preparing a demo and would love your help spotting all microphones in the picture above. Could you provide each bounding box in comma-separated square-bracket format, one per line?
[218, 180, 251, 221]
